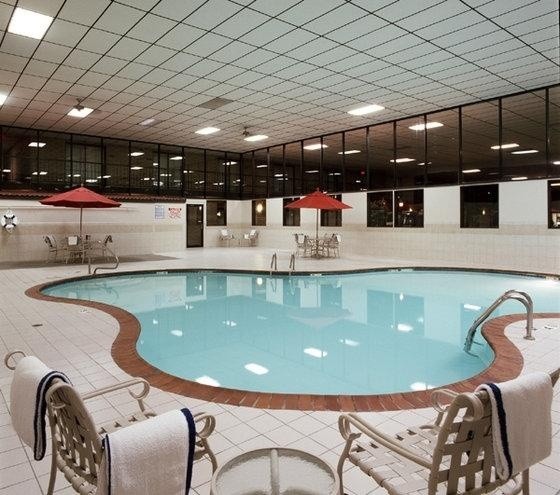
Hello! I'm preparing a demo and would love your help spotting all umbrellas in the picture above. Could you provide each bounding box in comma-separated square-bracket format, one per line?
[283, 188, 353, 259]
[39, 184, 122, 258]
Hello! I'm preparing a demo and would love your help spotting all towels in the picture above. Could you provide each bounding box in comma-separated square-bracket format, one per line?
[95, 407, 196, 495]
[464, 373, 555, 480]
[5, 351, 88, 463]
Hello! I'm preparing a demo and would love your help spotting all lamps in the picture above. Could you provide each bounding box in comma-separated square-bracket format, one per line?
[73, 97, 85, 112]
[242, 123, 252, 138]
[1, 207, 18, 234]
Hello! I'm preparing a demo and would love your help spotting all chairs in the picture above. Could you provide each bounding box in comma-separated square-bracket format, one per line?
[330, 366, 560, 495]
[293, 232, 343, 259]
[2, 348, 221, 495]
[41, 232, 115, 267]
[215, 228, 261, 247]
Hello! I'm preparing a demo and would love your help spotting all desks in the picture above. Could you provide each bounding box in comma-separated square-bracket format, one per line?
[209, 446, 342, 495]
